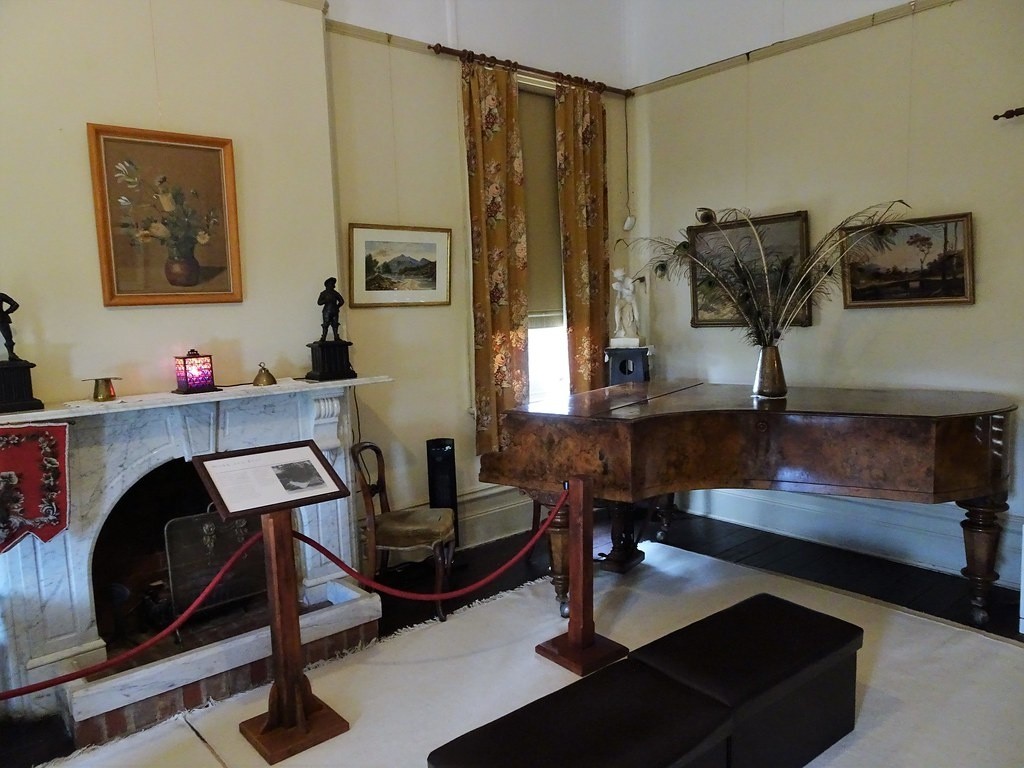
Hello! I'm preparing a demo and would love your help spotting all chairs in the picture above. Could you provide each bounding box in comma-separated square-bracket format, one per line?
[350, 442, 456, 622]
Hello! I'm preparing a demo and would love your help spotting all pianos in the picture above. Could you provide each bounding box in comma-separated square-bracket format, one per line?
[476, 380, 1018, 608]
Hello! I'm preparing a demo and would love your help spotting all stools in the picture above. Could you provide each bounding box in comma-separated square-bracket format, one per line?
[425, 659, 734, 768]
[626, 593, 864, 768]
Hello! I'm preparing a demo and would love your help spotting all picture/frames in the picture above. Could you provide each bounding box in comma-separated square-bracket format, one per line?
[87, 123, 243, 307]
[686, 210, 813, 327]
[839, 212, 975, 309]
[348, 223, 452, 309]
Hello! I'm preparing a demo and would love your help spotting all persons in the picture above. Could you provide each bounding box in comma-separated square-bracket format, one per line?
[317, 276, 344, 342]
[0, 292, 22, 362]
[611, 267, 641, 336]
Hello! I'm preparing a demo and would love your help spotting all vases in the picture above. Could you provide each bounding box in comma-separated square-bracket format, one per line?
[752, 346, 788, 398]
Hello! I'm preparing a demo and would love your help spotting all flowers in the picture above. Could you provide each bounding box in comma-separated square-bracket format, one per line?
[614, 198, 952, 343]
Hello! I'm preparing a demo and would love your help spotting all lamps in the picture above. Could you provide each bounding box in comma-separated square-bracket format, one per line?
[171, 349, 224, 395]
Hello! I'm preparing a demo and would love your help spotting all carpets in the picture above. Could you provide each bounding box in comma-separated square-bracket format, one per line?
[32, 539, 1024, 768]
